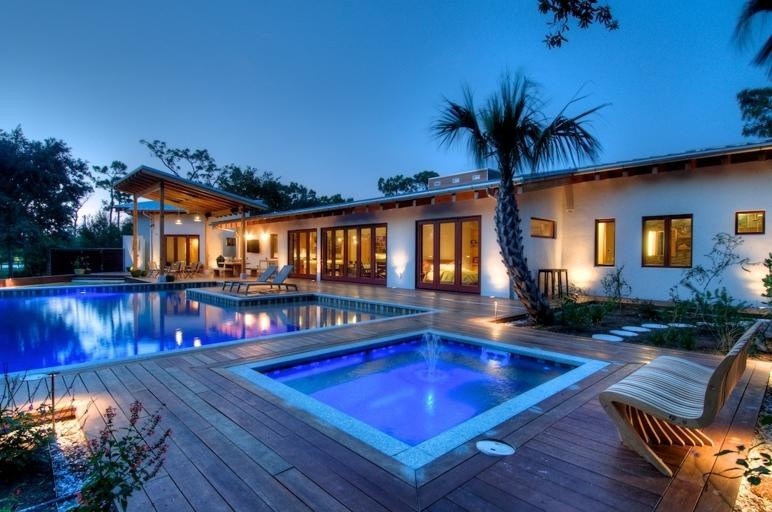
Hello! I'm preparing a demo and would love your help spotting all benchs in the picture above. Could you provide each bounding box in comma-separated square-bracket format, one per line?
[211, 268, 233, 277]
[598, 318, 764, 477]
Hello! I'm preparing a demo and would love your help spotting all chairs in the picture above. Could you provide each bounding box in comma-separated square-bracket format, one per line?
[222, 264, 279, 292]
[237, 265, 299, 296]
[148, 261, 199, 280]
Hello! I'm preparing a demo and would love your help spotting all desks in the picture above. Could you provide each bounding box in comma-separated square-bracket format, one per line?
[218, 262, 242, 275]
[537, 269, 569, 299]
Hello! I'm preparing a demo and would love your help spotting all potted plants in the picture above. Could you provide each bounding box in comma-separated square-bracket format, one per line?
[73, 256, 86, 275]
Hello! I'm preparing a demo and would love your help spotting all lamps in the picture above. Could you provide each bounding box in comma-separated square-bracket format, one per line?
[176, 204, 182, 224]
[194, 204, 201, 222]
